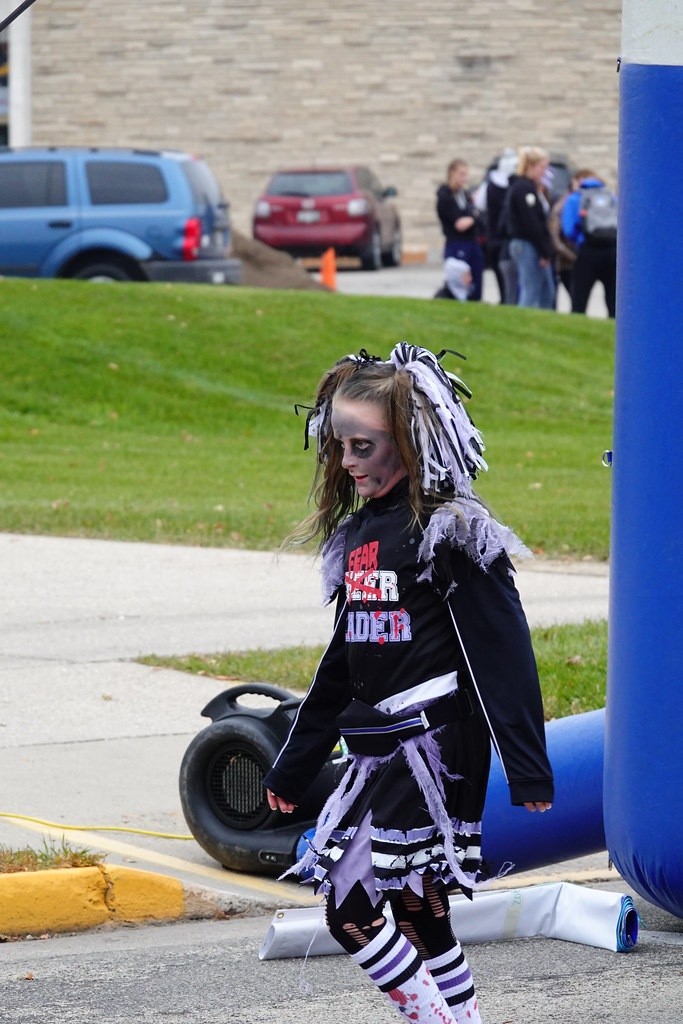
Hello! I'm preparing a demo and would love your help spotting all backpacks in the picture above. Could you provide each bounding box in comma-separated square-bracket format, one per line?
[572, 184, 618, 239]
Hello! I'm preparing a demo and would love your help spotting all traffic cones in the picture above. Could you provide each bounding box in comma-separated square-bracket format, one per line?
[319, 248, 339, 292]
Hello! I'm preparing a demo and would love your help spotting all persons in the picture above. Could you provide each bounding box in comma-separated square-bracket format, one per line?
[435, 146, 617, 318]
[264, 342, 554, 1024]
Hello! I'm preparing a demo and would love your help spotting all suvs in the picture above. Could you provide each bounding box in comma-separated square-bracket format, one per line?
[249, 163, 408, 272]
[0, 141, 241, 288]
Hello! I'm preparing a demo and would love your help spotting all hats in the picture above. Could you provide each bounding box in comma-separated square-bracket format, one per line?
[443, 257, 470, 288]
